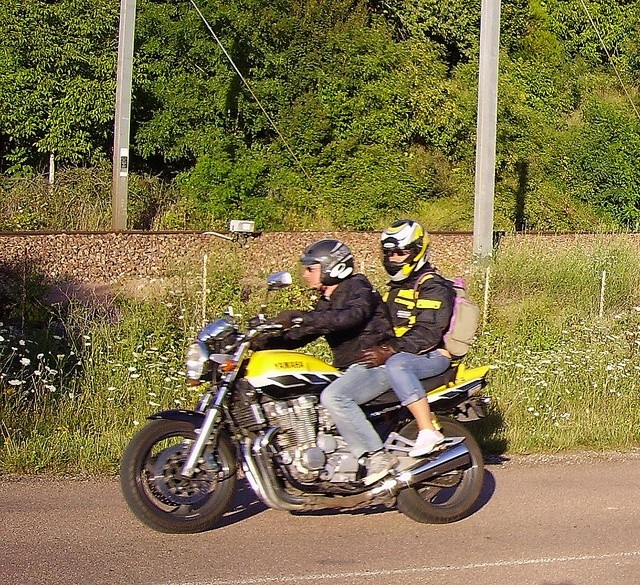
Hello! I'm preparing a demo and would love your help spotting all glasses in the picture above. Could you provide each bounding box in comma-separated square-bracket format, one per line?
[306, 267, 320, 272]
[385, 249, 410, 258]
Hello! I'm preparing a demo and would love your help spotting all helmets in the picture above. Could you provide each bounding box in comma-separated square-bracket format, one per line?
[380, 219, 432, 282]
[299, 238, 354, 285]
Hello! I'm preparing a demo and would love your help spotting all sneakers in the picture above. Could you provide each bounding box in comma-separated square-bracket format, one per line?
[365, 452, 397, 486]
[410, 429, 446, 458]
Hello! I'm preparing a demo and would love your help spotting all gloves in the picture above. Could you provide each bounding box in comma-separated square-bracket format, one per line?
[276, 310, 307, 326]
[358, 344, 393, 369]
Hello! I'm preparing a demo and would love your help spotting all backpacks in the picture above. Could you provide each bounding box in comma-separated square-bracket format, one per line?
[413, 272, 481, 356]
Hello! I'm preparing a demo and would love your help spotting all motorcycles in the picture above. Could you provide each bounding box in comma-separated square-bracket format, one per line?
[119, 271, 490, 532]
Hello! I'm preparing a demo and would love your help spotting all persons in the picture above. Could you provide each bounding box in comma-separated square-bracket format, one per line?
[362, 219, 457, 457]
[252, 240, 398, 488]
